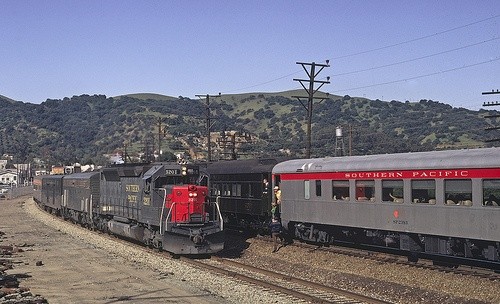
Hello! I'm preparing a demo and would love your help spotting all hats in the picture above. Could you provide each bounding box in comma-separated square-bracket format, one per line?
[274, 186, 279, 189]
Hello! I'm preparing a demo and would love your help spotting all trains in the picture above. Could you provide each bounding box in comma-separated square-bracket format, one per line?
[272, 147, 500, 270]
[32, 159, 280, 259]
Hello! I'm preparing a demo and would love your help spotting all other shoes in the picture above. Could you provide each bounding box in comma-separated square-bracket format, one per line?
[275, 217, 278, 220]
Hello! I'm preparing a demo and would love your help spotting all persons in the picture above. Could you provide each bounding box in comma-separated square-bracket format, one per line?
[273, 186, 282, 204]
[264, 178, 269, 189]
